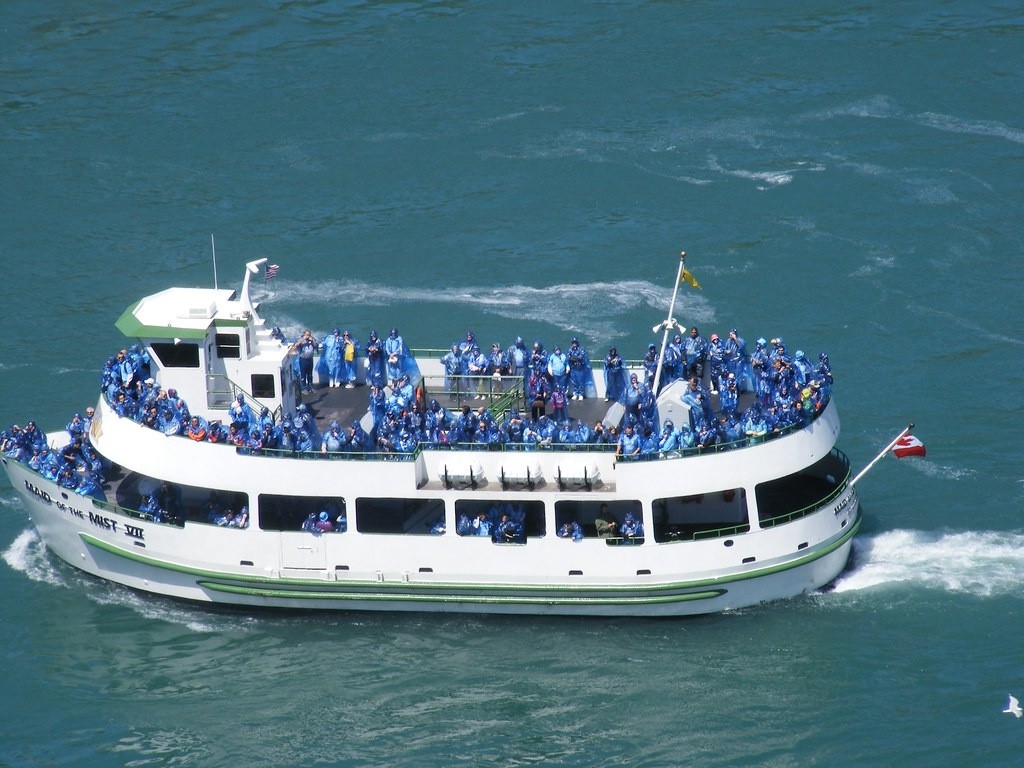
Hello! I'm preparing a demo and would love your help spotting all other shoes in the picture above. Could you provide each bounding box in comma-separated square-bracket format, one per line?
[309, 388, 318, 393]
[571, 395, 578, 400]
[604, 398, 609, 402]
[329, 380, 334, 388]
[452, 394, 501, 402]
[334, 382, 341, 388]
[578, 395, 583, 402]
[345, 382, 356, 389]
[301, 389, 309, 396]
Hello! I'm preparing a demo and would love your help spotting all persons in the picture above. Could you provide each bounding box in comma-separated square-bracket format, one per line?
[0, 326, 834, 546]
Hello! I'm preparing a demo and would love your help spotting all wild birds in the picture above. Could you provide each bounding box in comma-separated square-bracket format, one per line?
[1002, 694, 1023, 718]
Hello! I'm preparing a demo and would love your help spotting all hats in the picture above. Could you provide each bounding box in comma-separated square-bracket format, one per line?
[808, 380, 821, 388]
[819, 352, 828, 361]
[711, 333, 719, 342]
[757, 338, 767, 347]
[492, 343, 499, 349]
[144, 377, 154, 385]
[728, 373, 735, 378]
[801, 388, 811, 399]
[666, 422, 672, 425]
[795, 350, 805, 361]
[318, 511, 328, 522]
[572, 338, 578, 342]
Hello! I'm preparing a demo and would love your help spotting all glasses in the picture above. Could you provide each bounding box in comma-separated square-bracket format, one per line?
[480, 426, 485, 428]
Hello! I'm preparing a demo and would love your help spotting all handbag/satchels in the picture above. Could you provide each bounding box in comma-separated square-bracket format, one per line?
[531, 396, 545, 408]
[616, 449, 623, 462]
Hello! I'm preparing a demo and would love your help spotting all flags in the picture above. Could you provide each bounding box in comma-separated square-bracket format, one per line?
[265, 264, 280, 278]
[886, 433, 927, 459]
[681, 267, 702, 289]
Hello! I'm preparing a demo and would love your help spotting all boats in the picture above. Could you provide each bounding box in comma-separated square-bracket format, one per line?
[1, 256, 867, 620]
